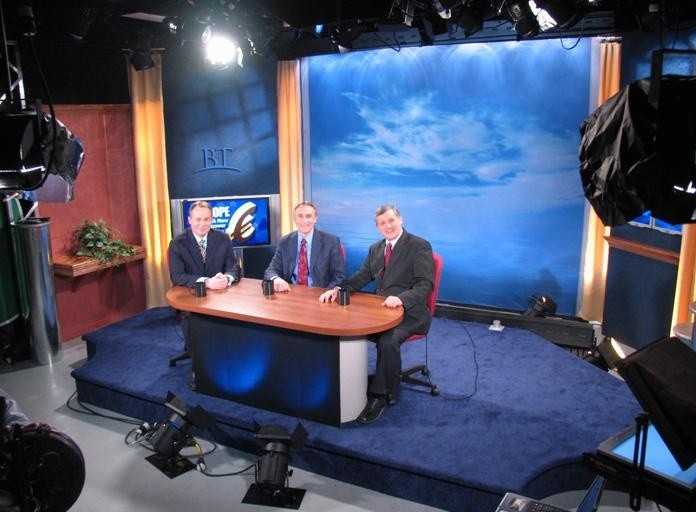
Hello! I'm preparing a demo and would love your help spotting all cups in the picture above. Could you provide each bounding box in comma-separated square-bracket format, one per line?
[189, 282, 206, 297]
[262, 280, 274, 295]
[337, 288, 350, 306]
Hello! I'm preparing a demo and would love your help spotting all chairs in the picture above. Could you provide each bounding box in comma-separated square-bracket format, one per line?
[386, 253, 442, 404]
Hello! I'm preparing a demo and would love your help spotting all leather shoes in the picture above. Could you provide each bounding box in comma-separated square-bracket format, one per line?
[357, 398, 385, 423]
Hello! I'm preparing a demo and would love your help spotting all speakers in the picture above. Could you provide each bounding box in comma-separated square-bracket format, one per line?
[639, 74, 696, 225]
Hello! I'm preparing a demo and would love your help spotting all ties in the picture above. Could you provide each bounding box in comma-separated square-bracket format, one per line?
[298, 239, 308, 286]
[199, 240, 207, 264]
[379, 243, 392, 280]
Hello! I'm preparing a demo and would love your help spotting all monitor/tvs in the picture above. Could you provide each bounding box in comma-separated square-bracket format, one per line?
[171, 194, 281, 250]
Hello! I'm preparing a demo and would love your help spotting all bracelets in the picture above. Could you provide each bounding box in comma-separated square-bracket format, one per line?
[225, 276, 230, 286]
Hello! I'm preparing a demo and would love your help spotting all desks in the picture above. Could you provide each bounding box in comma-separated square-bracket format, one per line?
[168, 276, 407, 427]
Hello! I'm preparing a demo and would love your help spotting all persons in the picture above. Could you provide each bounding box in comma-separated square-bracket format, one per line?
[263, 201, 345, 295]
[166, 200, 241, 355]
[317, 202, 437, 425]
[0, 382, 61, 512]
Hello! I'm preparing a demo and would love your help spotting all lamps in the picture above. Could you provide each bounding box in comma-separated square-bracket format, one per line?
[385, 1, 455, 28]
[521, 289, 557, 319]
[124, 390, 313, 510]
[129, 48, 152, 72]
[505, 1, 538, 44]
